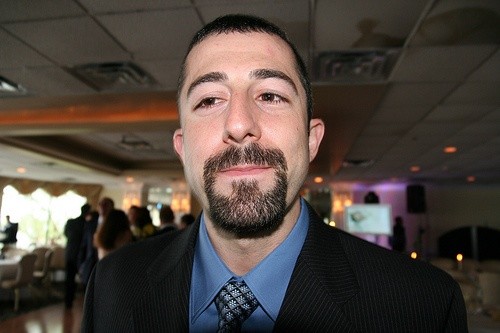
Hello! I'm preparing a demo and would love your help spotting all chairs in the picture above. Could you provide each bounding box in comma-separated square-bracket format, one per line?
[0, 246, 65, 311]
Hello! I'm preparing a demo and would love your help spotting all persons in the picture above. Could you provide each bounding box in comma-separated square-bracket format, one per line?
[389, 216, 430, 259]
[64, 198, 195, 309]
[0, 216, 12, 262]
[78, 14, 469, 333]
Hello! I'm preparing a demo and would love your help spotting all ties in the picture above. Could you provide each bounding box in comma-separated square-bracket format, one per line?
[214, 280, 259, 333]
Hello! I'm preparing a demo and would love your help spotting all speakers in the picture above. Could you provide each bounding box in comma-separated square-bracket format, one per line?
[407, 184, 425, 214]
